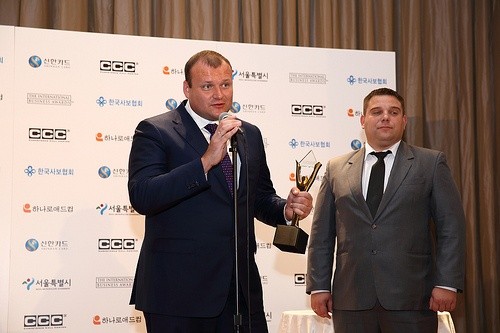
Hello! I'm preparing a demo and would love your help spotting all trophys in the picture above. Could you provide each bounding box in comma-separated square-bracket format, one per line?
[273, 149, 322, 255]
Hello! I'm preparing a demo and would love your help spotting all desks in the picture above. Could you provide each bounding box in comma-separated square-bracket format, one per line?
[282, 310, 455, 333]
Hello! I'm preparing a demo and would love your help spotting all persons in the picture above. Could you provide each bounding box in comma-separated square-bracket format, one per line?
[306, 88, 467, 333]
[128, 51, 313, 333]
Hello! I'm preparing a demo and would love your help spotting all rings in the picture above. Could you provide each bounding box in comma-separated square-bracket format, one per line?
[314, 309, 317, 312]
[218, 130, 223, 136]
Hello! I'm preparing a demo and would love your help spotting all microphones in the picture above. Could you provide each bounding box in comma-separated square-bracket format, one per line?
[219, 112, 245, 135]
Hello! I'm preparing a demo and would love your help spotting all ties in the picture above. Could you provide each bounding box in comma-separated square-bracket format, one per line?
[366, 151, 392, 219]
[205, 124, 233, 201]
